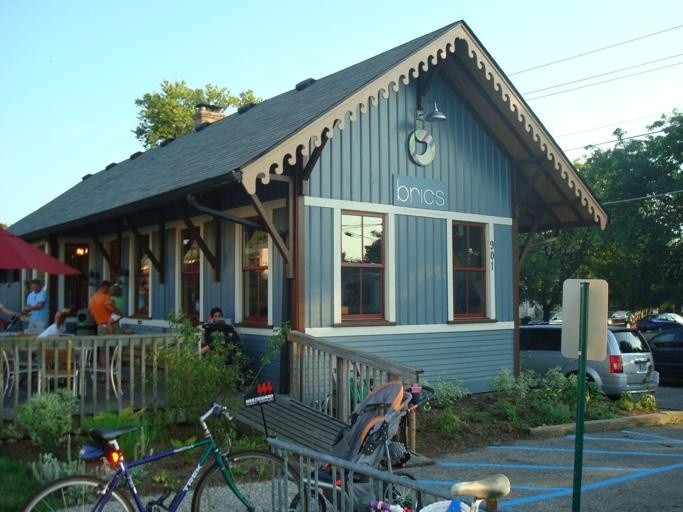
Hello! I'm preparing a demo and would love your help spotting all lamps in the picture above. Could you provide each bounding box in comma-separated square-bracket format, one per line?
[417, 100, 446, 123]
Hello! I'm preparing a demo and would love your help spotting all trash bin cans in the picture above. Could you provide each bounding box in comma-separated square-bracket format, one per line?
[75, 309, 98, 366]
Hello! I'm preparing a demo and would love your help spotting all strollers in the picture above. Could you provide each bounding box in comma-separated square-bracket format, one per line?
[290, 381, 437, 512]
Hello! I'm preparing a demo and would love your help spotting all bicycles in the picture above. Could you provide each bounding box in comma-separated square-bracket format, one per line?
[20, 402, 309, 512]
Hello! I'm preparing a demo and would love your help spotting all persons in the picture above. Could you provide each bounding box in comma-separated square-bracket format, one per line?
[16, 277, 50, 334]
[35, 307, 72, 340]
[0, 302, 21, 332]
[87, 277, 123, 383]
[193, 306, 245, 392]
[100, 284, 124, 334]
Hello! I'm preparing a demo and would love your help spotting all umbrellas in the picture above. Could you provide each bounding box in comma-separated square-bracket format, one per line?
[0, 224, 83, 276]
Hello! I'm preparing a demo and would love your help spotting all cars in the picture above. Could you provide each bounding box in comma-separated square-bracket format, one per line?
[519, 310, 683, 396]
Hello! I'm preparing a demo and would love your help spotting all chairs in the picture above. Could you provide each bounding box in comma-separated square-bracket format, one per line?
[1, 328, 125, 401]
